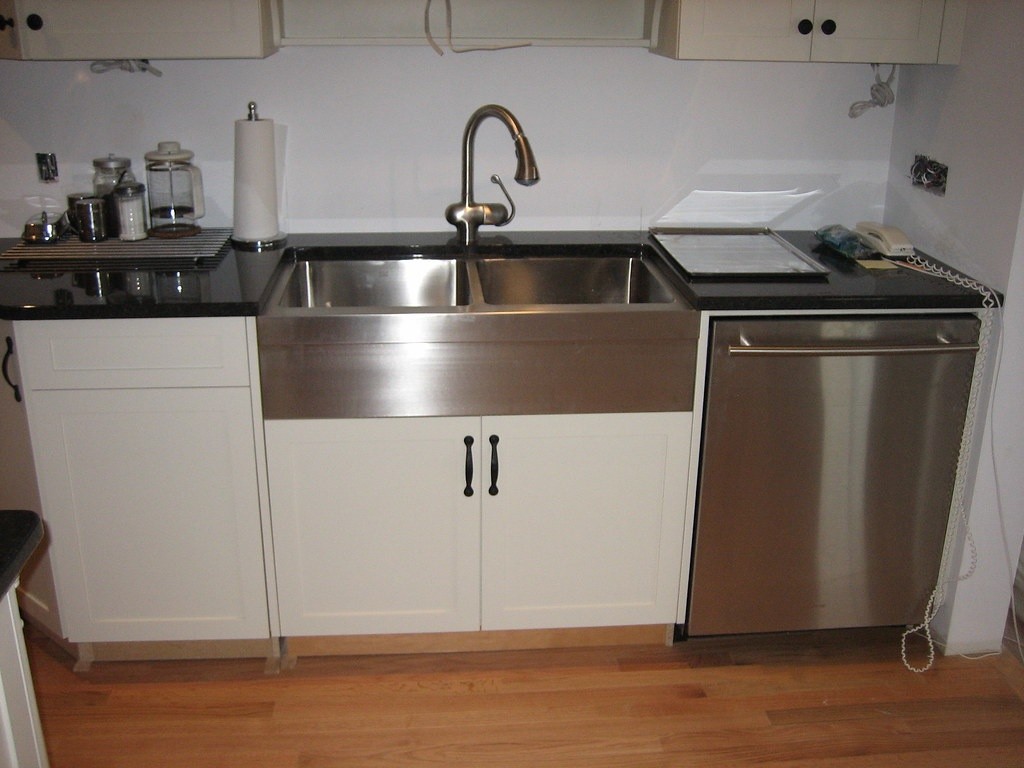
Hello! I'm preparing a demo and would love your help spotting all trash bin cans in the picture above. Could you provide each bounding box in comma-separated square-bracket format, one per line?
[0, 509, 51, 768]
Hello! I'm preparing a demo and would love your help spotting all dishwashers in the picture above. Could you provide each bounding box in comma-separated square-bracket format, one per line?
[686, 319, 983, 638]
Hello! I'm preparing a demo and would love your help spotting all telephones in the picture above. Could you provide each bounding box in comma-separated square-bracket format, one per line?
[855, 220, 916, 257]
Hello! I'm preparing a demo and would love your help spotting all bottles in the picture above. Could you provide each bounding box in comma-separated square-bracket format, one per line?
[112, 170, 148, 241]
[92, 153, 135, 237]
[67, 192, 106, 242]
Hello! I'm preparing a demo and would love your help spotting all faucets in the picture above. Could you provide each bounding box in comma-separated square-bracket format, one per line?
[447, 100, 545, 241]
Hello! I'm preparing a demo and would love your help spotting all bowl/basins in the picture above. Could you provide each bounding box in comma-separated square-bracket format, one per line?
[21, 211, 70, 243]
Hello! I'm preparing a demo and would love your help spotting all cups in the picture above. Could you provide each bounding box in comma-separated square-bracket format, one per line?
[144, 142, 206, 237]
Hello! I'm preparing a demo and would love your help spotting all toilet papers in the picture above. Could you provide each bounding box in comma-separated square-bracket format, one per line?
[231, 117, 279, 241]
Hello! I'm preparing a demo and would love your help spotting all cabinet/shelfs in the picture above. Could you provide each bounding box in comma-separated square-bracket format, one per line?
[272, 0, 662, 49]
[258, 413, 690, 656]
[652, 0, 969, 66]
[0, 0, 278, 61]
[11, 318, 269, 672]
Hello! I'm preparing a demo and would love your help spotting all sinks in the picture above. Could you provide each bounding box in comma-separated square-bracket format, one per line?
[253, 241, 475, 316]
[472, 236, 694, 317]
